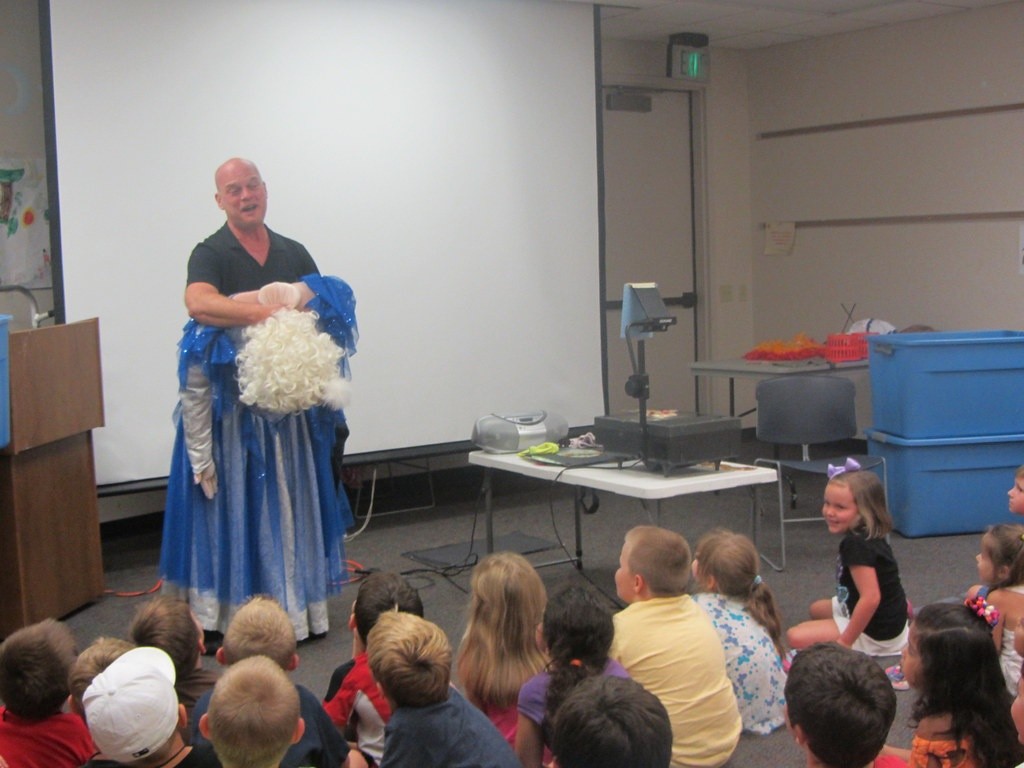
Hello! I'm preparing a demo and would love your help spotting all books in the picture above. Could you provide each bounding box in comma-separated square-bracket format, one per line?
[525, 447, 638, 467]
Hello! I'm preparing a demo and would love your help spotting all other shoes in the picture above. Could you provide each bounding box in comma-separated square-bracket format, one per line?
[781, 649, 797, 673]
[202, 630, 223, 654]
[886, 665, 909, 690]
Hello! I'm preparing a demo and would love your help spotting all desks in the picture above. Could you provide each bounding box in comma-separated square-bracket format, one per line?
[688, 357, 870, 508]
[467, 450, 779, 609]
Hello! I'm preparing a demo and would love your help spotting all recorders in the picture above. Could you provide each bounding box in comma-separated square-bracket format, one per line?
[471, 412, 569, 453]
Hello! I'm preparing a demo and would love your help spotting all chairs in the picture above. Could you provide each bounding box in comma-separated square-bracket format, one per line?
[752, 374, 889, 572]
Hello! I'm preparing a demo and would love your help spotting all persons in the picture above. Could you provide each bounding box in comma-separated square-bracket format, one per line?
[881, 597, 1024, 768]
[189, 596, 368, 768]
[1010, 661, 1024, 768]
[608, 524, 743, 768]
[514, 585, 630, 768]
[0, 617, 221, 768]
[458, 552, 555, 768]
[322, 571, 424, 768]
[366, 611, 524, 768]
[160, 157, 360, 655]
[783, 642, 913, 768]
[885, 524, 1024, 696]
[199, 655, 305, 768]
[688, 532, 789, 735]
[966, 464, 1024, 602]
[129, 594, 221, 745]
[787, 457, 913, 657]
[552, 674, 673, 768]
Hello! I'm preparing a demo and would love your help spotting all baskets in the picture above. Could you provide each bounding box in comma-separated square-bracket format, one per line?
[853, 332, 877, 357]
[824, 333, 862, 360]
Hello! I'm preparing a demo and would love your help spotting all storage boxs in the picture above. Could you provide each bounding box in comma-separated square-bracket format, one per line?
[864, 331, 1024, 538]
[595, 412, 740, 466]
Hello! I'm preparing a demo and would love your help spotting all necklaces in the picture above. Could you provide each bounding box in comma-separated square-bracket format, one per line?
[156, 745, 187, 768]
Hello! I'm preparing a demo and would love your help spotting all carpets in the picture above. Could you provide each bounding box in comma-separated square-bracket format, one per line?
[403, 530, 564, 570]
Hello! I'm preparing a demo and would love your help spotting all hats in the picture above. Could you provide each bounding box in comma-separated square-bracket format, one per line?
[81, 646, 180, 763]
